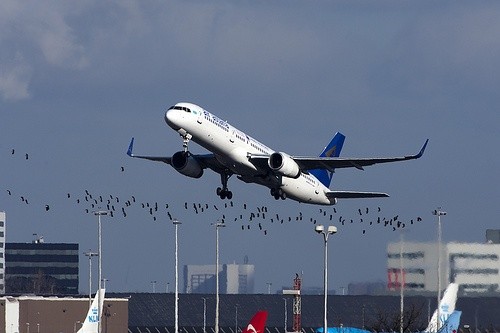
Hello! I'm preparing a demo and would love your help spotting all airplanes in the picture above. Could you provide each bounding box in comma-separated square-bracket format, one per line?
[126, 102, 429, 205]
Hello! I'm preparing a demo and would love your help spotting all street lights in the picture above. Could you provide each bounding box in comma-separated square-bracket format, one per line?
[85, 253, 99, 310]
[431, 209, 447, 332]
[172, 220, 182, 332]
[94, 210, 107, 333]
[209, 222, 227, 332]
[314, 224, 338, 333]
